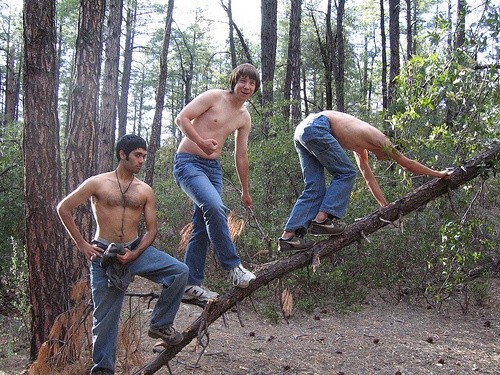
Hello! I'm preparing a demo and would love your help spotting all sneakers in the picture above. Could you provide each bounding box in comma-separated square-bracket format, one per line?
[309, 217, 348, 237]
[224, 261, 257, 289]
[277, 234, 317, 253]
[180, 283, 221, 304]
[147, 323, 186, 347]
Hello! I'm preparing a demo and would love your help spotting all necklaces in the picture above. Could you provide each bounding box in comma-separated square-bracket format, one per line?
[116, 169, 134, 242]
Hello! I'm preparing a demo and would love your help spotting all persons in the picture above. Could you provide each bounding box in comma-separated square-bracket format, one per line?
[175, 63, 260, 303]
[56, 134, 189, 375]
[278, 111, 454, 252]
